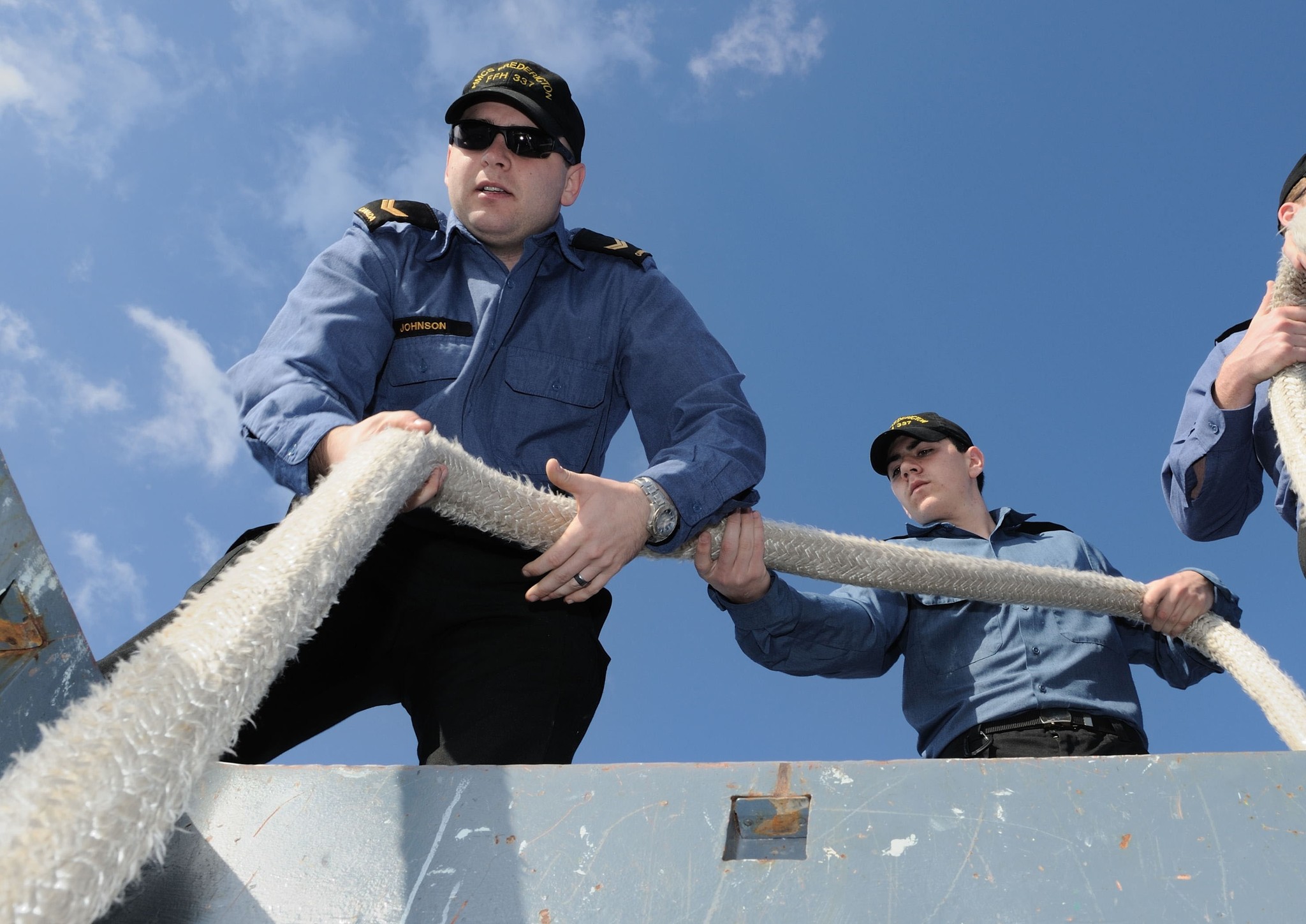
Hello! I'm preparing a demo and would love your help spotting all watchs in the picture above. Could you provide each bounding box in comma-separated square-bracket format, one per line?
[630, 476, 678, 544]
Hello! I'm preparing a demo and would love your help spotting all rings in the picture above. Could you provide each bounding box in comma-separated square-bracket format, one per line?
[573, 574, 590, 588]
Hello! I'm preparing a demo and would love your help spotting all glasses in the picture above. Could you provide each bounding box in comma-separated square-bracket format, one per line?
[449, 119, 577, 165]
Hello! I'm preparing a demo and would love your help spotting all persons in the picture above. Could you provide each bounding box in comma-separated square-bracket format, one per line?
[1162, 150, 1305, 576]
[695, 413, 1244, 758]
[96, 59, 768, 766]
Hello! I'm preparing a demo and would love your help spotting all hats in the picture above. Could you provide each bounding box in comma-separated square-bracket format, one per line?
[1278, 153, 1306, 236]
[444, 58, 586, 164]
[870, 412, 974, 476]
